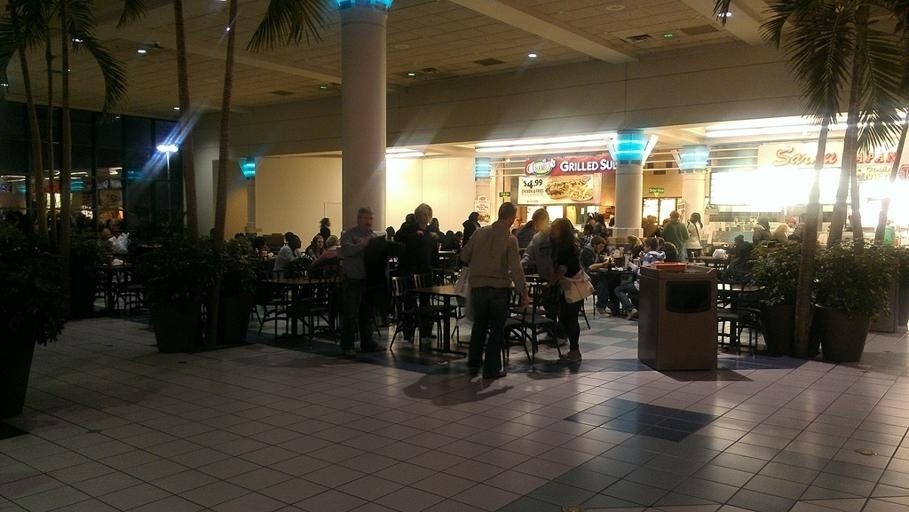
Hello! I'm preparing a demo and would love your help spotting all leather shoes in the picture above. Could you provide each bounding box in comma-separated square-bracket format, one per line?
[470, 366, 479, 374]
[483, 372, 506, 379]
[539, 336, 568, 347]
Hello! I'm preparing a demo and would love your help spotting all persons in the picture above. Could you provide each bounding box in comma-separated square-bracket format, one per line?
[237, 217, 342, 319]
[209, 228, 229, 244]
[342, 203, 464, 358]
[462, 203, 530, 378]
[519, 220, 584, 374]
[717, 214, 806, 281]
[101, 227, 117, 245]
[579, 206, 704, 319]
[515, 208, 549, 258]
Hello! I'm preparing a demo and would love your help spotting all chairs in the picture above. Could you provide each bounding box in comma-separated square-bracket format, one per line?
[94, 251, 148, 316]
[718, 275, 763, 357]
[251, 248, 637, 370]
[686, 249, 732, 278]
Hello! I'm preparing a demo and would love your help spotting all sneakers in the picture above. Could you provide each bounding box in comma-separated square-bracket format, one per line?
[342, 349, 356, 356]
[627, 308, 638, 319]
[363, 344, 387, 352]
[560, 344, 581, 361]
[595, 308, 610, 318]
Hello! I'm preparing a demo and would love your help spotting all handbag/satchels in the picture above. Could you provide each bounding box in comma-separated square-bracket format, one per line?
[561, 269, 594, 304]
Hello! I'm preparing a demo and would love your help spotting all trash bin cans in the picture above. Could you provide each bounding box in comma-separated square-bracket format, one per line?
[638, 262, 718, 371]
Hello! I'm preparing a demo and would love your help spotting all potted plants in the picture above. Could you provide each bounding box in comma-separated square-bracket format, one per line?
[0, 207, 70, 419]
[745, 240, 823, 358]
[814, 237, 897, 363]
[895, 245, 909, 326]
